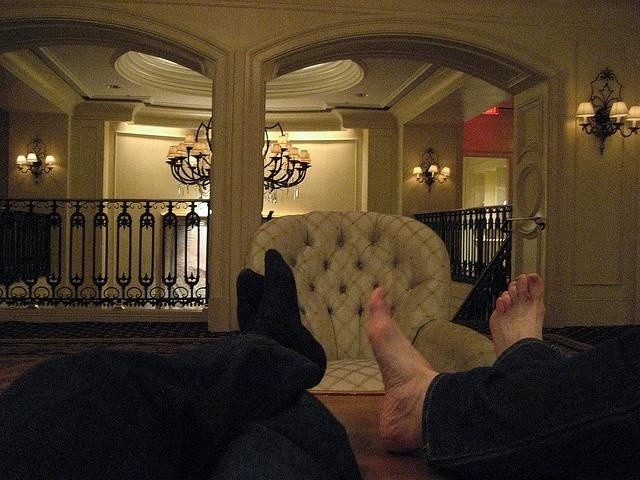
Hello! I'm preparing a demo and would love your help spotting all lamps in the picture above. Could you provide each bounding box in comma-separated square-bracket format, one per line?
[163, 112, 312, 198]
[574, 68, 640, 155]
[412, 147, 451, 186]
[15, 135, 56, 178]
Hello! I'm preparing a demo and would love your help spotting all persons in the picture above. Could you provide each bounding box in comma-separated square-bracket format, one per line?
[1, 250, 362, 480]
[367, 270, 640, 480]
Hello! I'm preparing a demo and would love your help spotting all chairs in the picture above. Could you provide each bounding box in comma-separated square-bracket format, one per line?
[239, 210, 496, 399]
